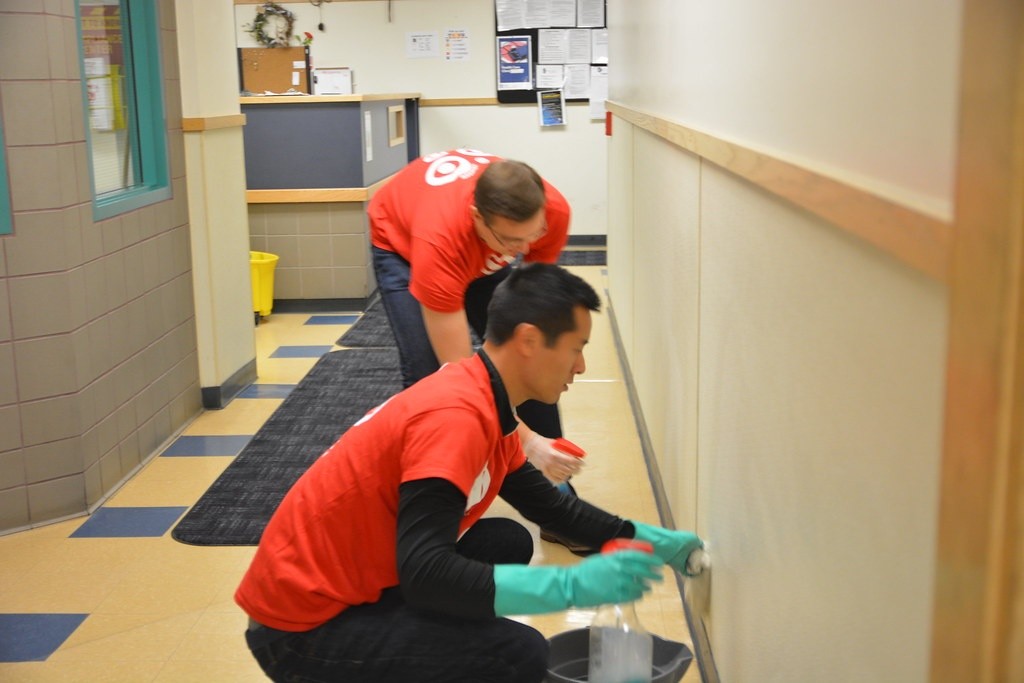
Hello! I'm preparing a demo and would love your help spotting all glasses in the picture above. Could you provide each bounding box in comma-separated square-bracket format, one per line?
[485, 220, 549, 251]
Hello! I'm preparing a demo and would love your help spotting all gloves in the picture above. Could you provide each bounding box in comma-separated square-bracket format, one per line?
[492, 551, 667, 620]
[622, 515, 706, 577]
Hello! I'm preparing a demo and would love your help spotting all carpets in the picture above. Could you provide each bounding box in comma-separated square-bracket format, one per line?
[172, 249, 607, 547]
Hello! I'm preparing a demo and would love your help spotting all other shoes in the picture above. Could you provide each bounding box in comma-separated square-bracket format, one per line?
[539, 525, 594, 556]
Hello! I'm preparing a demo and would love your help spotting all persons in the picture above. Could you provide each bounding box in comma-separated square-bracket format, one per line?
[235, 261, 704, 683]
[368, 151, 598, 556]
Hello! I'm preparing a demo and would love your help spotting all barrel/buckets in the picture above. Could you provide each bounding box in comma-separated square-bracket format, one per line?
[544, 626, 693, 683]
